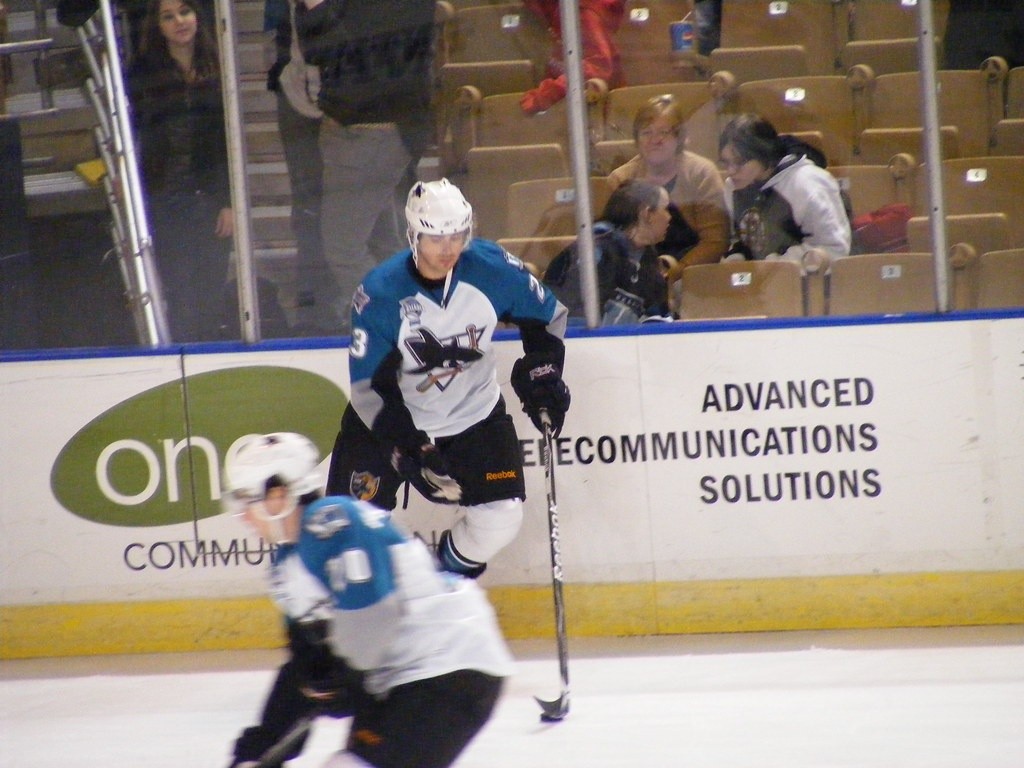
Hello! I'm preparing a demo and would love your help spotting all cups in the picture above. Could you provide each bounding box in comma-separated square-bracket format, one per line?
[670, 21, 694, 48]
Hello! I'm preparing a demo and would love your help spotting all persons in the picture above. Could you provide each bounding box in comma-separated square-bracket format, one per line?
[233, 177, 574, 768]
[518, 0, 627, 113]
[113, 0, 236, 344]
[213, 428, 517, 768]
[543, 175, 681, 329]
[718, 118, 852, 310]
[691, 0, 723, 58]
[943, 0, 1024, 72]
[600, 93, 733, 309]
[263, 0, 453, 341]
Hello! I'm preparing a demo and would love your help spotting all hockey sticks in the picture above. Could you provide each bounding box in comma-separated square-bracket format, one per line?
[261, 707, 321, 763]
[528, 407, 574, 723]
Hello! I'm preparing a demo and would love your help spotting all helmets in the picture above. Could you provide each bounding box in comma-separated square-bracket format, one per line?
[223, 428, 321, 501]
[404, 176, 473, 249]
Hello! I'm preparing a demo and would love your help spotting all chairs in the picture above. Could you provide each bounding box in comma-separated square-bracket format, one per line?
[430, 0, 1024, 333]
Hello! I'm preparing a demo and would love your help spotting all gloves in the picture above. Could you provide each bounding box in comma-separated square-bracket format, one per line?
[295, 659, 360, 721]
[371, 407, 461, 504]
[511, 348, 570, 434]
[230, 713, 308, 768]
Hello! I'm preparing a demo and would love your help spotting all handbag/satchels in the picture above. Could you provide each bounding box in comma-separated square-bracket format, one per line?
[851, 201, 912, 255]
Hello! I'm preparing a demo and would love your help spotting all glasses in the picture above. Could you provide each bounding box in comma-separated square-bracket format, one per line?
[716, 158, 753, 169]
